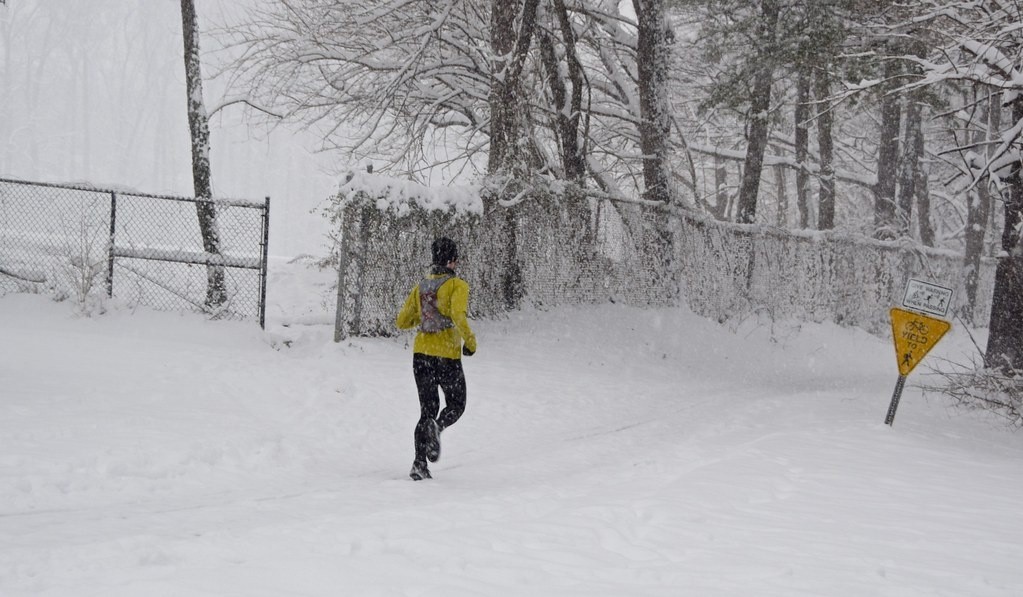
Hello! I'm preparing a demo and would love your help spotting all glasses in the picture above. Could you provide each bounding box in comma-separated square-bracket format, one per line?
[452, 254, 459, 262]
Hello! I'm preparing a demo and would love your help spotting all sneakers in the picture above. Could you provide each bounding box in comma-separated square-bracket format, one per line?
[423, 419, 440, 461]
[409, 460, 432, 480]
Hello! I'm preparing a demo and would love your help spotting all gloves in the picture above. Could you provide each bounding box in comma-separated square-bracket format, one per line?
[463, 346, 475, 356]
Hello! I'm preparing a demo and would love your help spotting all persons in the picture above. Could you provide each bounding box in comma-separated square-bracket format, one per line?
[395, 236, 476, 481]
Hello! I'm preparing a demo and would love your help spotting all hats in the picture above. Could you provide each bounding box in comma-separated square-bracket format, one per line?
[431, 237, 456, 265]
